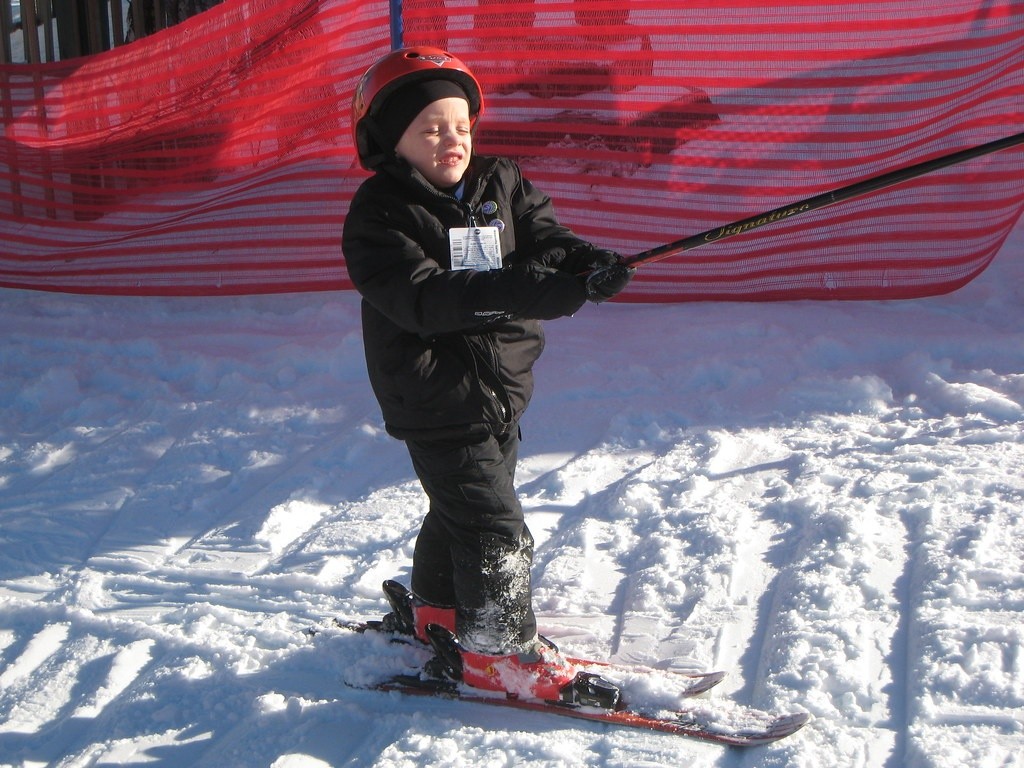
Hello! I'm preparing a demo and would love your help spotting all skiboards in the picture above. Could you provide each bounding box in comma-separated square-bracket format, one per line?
[307, 618, 726, 697]
[343, 664, 810, 748]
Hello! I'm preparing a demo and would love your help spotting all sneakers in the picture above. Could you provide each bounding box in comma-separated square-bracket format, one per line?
[424, 621, 620, 710]
[383, 580, 560, 656]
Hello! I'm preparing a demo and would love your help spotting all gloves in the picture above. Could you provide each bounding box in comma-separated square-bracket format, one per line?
[494, 237, 637, 319]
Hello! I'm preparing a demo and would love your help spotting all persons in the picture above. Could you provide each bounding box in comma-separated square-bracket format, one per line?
[340, 43, 637, 710]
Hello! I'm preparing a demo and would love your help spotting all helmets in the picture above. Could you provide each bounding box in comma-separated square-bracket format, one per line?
[351, 46, 485, 171]
